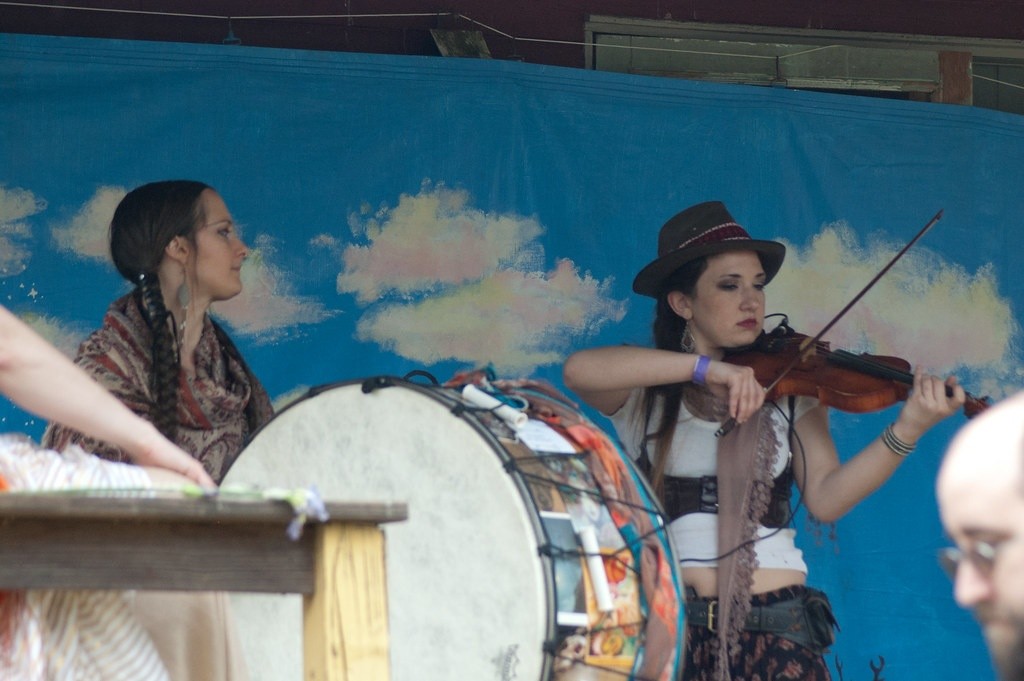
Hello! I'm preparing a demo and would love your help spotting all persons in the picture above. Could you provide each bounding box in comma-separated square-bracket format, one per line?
[43, 179, 273, 493]
[566, 200, 965, 681]
[0, 302, 248, 681]
[937, 392, 1024, 681]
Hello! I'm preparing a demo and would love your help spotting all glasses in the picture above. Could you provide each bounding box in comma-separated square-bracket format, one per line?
[940, 541, 1000, 574]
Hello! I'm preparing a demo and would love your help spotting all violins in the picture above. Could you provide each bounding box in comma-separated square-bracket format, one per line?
[722, 331, 989, 421]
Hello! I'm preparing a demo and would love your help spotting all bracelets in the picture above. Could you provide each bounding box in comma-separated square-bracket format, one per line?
[882, 425, 916, 455]
[692, 355, 711, 386]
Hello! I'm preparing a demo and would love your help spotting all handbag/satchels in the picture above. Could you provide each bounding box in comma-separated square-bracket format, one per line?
[685, 591, 840, 656]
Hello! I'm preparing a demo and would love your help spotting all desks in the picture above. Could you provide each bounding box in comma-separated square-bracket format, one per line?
[0, 493, 411, 681]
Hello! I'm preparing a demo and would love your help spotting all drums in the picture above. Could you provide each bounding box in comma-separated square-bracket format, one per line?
[217, 376, 687, 680]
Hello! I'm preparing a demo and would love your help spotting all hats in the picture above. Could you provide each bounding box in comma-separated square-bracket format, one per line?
[633, 201, 787, 298]
